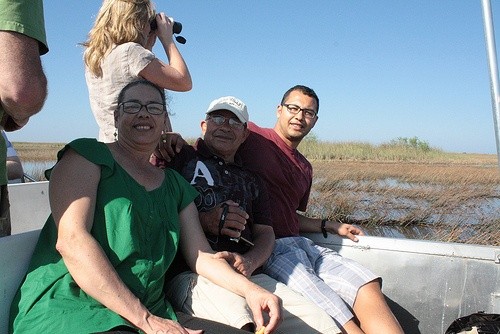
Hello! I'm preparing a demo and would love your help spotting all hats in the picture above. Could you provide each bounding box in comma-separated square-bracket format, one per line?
[205, 96, 248, 124]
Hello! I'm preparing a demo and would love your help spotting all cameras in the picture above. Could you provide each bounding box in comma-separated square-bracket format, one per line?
[229, 199, 256, 249]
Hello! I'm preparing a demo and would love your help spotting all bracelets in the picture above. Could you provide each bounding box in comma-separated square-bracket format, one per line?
[321, 219, 328, 238]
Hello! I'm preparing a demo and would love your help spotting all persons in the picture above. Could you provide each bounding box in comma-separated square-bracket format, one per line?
[0, 0, 49, 239]
[9, 79, 283, 334]
[153, 85, 405, 334]
[82, 0, 193, 143]
[155, 96, 343, 334]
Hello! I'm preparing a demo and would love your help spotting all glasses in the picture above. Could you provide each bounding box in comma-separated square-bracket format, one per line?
[118, 100, 165, 115]
[208, 114, 246, 129]
[281, 103, 317, 119]
